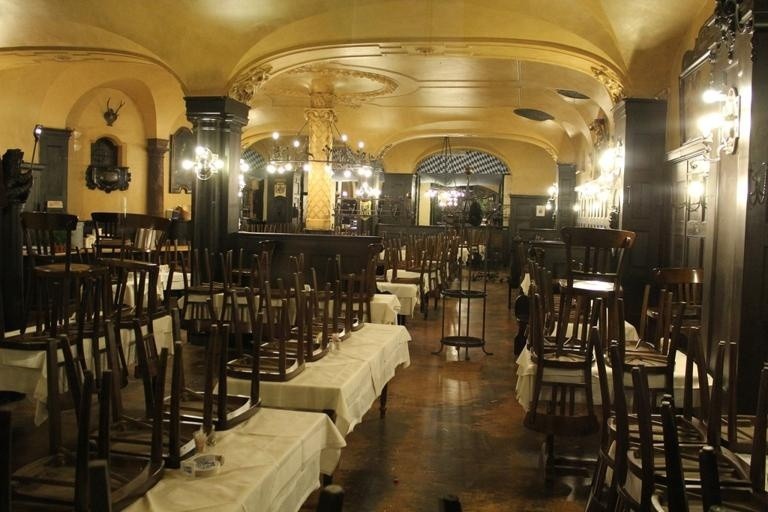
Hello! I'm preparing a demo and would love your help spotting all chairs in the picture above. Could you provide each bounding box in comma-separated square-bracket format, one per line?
[603, 289, 688, 413]
[99, 212, 171, 307]
[334, 253, 377, 303]
[2, 335, 171, 512]
[650, 399, 767, 511]
[22, 209, 93, 333]
[294, 252, 337, 313]
[93, 213, 159, 310]
[557, 225, 636, 361]
[242, 279, 332, 362]
[252, 251, 294, 307]
[580, 340, 728, 510]
[287, 267, 355, 340]
[507, 234, 532, 309]
[59, 318, 217, 468]
[226, 246, 252, 287]
[650, 266, 704, 340]
[389, 243, 437, 318]
[221, 286, 307, 384]
[132, 306, 264, 431]
[306, 266, 367, 332]
[523, 284, 602, 438]
[194, 246, 225, 288]
[154, 237, 191, 272]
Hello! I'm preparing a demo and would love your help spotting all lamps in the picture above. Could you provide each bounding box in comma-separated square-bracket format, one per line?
[28, 123, 46, 174]
[179, 120, 225, 181]
[680, 179, 703, 213]
[686, 82, 742, 181]
[424, 136, 466, 209]
[264, 86, 375, 179]
